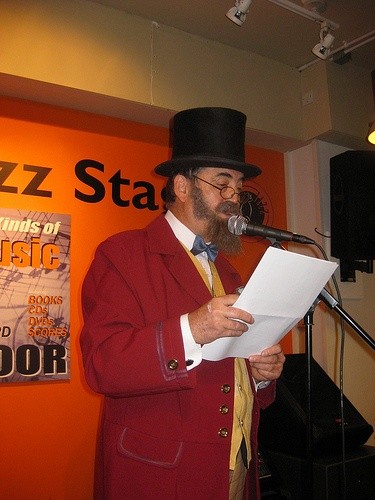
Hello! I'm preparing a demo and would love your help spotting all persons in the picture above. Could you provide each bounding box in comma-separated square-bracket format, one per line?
[79, 107, 286, 500]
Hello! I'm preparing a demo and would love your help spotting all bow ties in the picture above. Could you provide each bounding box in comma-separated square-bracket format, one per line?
[191, 236, 222, 262]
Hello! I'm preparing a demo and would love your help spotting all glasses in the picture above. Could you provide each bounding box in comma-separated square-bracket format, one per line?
[186, 175, 251, 201]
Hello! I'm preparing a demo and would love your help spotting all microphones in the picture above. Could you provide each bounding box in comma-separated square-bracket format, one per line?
[228, 215, 315, 244]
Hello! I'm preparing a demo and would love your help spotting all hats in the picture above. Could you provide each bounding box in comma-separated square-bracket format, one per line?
[151, 108, 265, 178]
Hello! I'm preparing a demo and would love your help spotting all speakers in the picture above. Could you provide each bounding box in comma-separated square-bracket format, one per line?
[330, 150, 375, 260]
[262, 352, 375, 500]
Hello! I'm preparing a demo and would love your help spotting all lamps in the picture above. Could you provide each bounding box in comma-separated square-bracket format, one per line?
[367, 120, 375, 145]
[225, 0, 336, 61]
